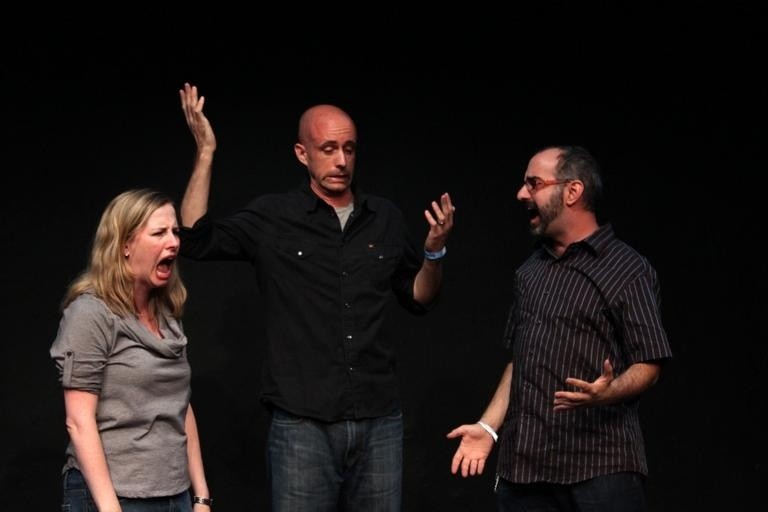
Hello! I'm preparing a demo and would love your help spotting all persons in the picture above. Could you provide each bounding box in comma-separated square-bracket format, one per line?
[446, 148, 674, 512]
[175, 81, 456, 512]
[48, 189, 214, 512]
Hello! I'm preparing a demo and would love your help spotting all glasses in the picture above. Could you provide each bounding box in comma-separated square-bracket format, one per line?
[526, 179, 565, 193]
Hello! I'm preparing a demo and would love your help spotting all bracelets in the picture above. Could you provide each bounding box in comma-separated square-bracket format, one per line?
[192, 497, 213, 507]
[424, 245, 447, 262]
[477, 419, 500, 443]
